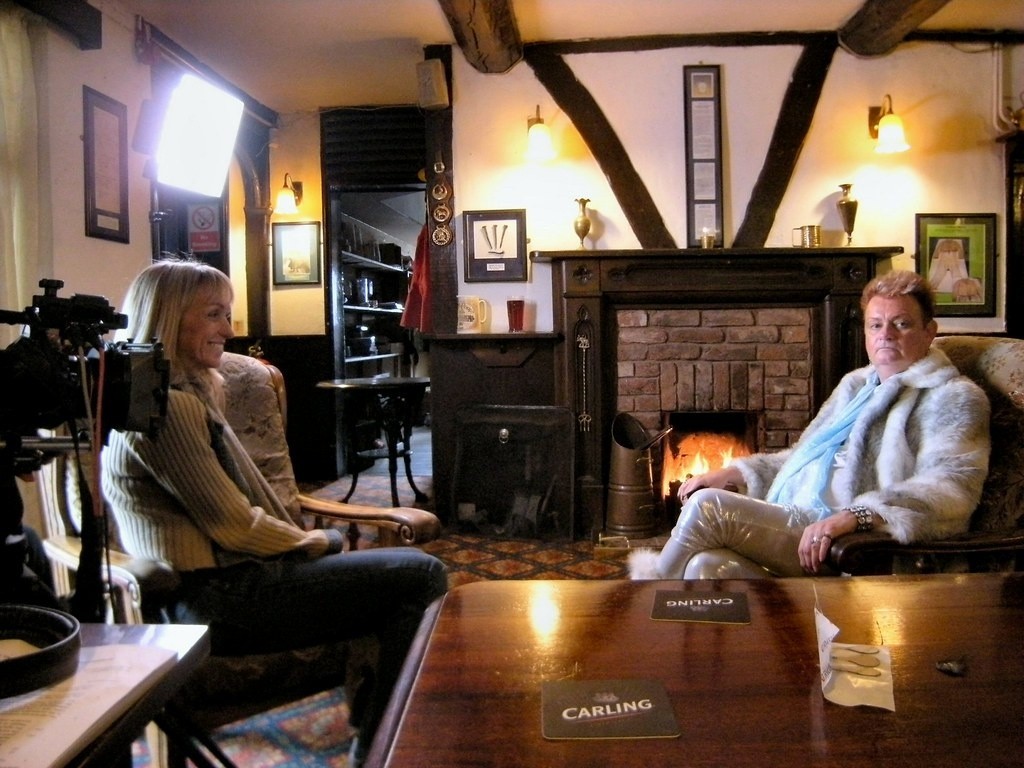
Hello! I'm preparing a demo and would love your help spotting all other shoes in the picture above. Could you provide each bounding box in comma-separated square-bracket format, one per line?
[349, 736, 372, 768]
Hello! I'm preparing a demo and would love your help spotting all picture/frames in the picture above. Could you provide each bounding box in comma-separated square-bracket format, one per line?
[81, 83, 130, 247]
[914, 210, 998, 318]
[461, 208, 530, 284]
[271, 222, 322, 285]
[680, 62, 727, 248]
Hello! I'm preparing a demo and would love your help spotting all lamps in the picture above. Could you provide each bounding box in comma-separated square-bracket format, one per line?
[868, 92, 911, 155]
[274, 169, 305, 217]
[125, 70, 249, 204]
[525, 104, 552, 156]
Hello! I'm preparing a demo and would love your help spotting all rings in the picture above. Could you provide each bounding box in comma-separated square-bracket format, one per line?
[811, 538, 821, 544]
[822, 534, 833, 540]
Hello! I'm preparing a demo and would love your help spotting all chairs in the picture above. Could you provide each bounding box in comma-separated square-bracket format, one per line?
[39, 351, 442, 768]
[820, 332, 1024, 576]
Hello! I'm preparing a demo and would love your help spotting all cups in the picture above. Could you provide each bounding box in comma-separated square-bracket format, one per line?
[506, 300, 525, 333]
[701, 236, 714, 248]
[791, 224, 822, 249]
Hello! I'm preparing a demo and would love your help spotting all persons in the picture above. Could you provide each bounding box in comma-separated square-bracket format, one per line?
[629, 272, 991, 580]
[97, 261, 446, 764]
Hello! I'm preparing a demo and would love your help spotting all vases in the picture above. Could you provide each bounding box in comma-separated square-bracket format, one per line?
[573, 198, 592, 250]
[835, 183, 859, 245]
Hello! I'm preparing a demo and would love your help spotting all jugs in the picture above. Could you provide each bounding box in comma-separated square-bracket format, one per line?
[456, 295, 486, 334]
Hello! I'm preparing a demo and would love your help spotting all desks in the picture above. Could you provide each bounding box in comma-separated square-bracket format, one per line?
[0, 624, 213, 768]
[361, 571, 1024, 768]
[319, 371, 431, 506]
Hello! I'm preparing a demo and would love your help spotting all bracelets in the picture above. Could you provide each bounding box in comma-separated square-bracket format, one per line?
[841, 504, 873, 532]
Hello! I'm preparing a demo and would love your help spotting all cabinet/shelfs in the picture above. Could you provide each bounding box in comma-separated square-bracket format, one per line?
[342, 212, 419, 375]
